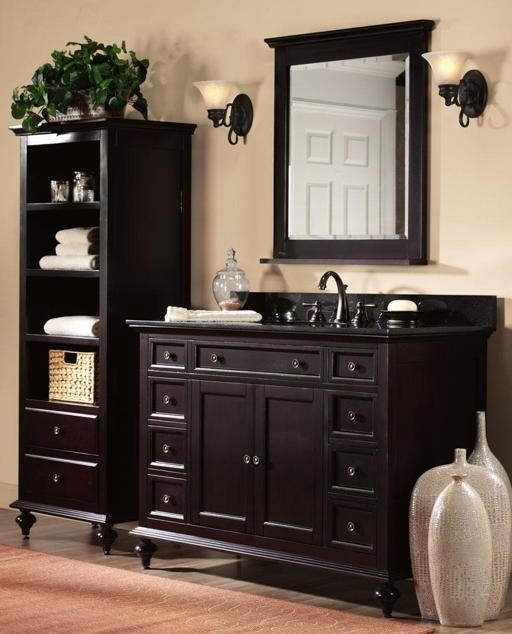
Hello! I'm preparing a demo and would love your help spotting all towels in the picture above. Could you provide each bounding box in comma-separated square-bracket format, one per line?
[165, 306, 262, 323]
[43, 315, 100, 338]
[55, 227, 99, 245]
[55, 245, 91, 255]
[38, 255, 99, 270]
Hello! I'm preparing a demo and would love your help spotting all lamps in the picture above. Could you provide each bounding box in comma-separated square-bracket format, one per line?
[191, 77, 254, 145]
[422, 44, 488, 127]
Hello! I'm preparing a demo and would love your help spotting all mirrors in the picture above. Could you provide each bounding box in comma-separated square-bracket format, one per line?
[264, 19, 434, 266]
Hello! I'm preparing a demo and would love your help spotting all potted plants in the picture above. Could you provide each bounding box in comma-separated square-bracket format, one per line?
[7, 34, 150, 135]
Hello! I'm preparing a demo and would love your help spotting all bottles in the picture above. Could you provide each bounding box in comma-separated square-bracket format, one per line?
[467, 411, 512, 512]
[212, 249, 250, 311]
[51, 180, 70, 202]
[72, 171, 94, 202]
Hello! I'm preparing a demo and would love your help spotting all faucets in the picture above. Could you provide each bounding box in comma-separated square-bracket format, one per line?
[317, 270, 350, 328]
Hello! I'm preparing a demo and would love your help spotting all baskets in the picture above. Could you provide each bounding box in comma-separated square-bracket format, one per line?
[44, 91, 127, 121]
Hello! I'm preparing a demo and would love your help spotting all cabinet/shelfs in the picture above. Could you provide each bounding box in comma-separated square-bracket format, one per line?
[9, 120, 197, 555]
[127, 319, 493, 618]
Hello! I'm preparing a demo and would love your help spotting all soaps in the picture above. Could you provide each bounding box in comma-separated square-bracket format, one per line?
[387, 299, 419, 311]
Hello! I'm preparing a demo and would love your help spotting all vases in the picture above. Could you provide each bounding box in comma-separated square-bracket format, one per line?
[465, 410, 512, 498]
